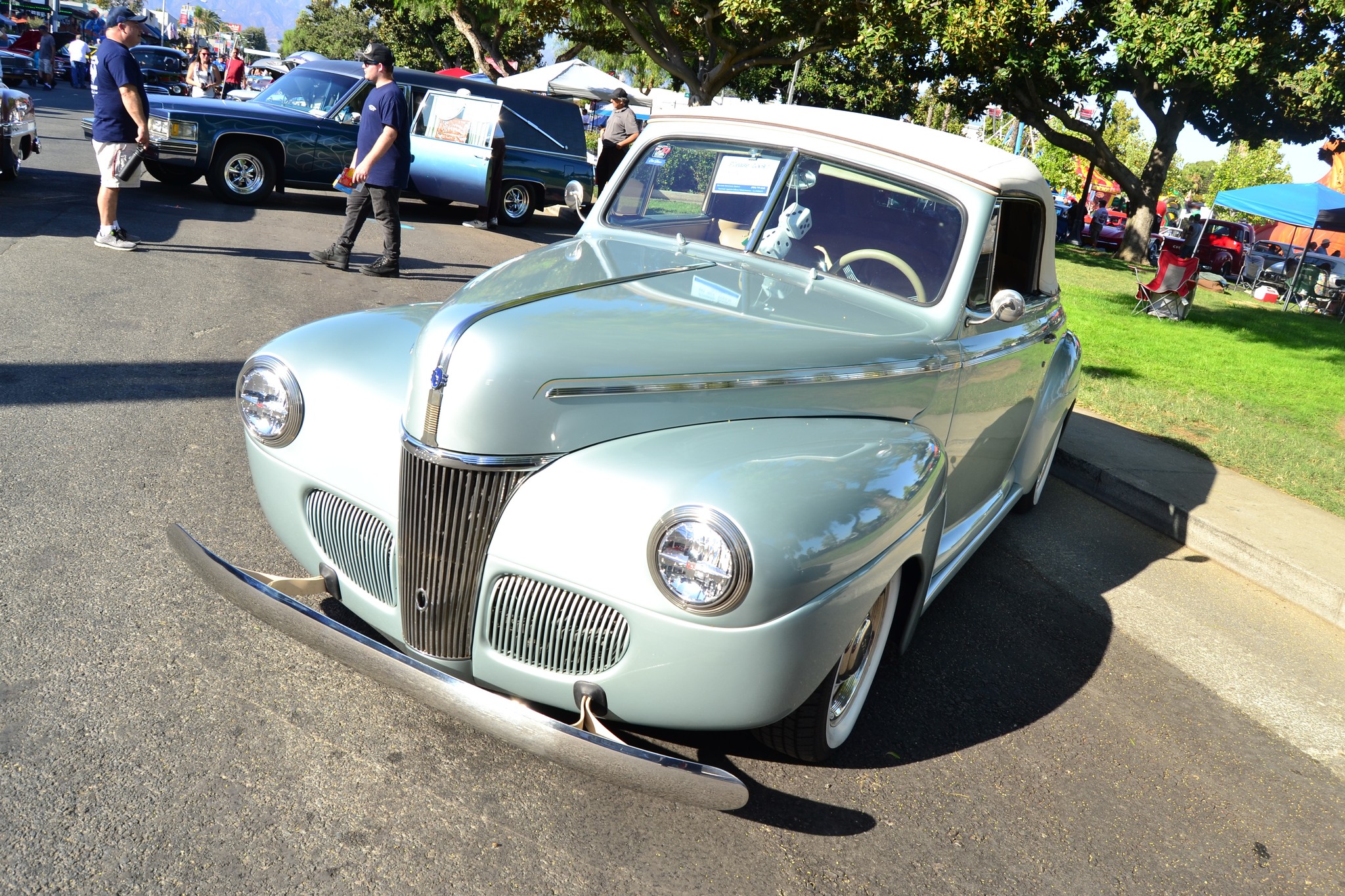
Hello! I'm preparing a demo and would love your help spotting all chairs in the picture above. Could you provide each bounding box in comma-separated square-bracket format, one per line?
[1126, 248, 1201, 324]
[1279, 257, 1345, 324]
[1233, 253, 1268, 296]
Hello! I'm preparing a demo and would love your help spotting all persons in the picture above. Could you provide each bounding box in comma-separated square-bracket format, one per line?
[1306, 242, 1318, 253]
[0, 16, 288, 105]
[1315, 239, 1331, 255]
[1315, 262, 1343, 316]
[1267, 244, 1278, 255]
[1180, 215, 1196, 239]
[1286, 257, 1300, 279]
[1179, 214, 1203, 258]
[1331, 250, 1341, 257]
[1052, 194, 1120, 243]
[307, 42, 413, 278]
[596, 87, 641, 202]
[1089, 199, 1108, 249]
[88, 6, 149, 252]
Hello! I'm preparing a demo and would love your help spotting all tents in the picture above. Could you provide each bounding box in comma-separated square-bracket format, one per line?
[435, 67, 495, 85]
[495, 58, 656, 143]
[1190, 182, 1345, 313]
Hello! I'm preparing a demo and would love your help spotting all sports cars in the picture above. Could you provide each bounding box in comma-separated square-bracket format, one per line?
[1079, 215, 1164, 261]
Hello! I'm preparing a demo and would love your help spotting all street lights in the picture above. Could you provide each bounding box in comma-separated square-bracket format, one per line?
[226, 31, 255, 64]
[217, 25, 232, 59]
[185, 0, 207, 34]
[196, 9, 226, 43]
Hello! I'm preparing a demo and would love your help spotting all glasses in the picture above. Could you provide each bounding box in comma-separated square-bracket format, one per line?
[363, 58, 386, 67]
[1327, 268, 1331, 272]
[199, 53, 210, 56]
[1313, 244, 1317, 247]
[1336, 254, 1341, 256]
[233, 52, 239, 54]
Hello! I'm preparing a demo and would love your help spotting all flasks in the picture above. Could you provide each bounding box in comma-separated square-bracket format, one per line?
[118, 142, 146, 181]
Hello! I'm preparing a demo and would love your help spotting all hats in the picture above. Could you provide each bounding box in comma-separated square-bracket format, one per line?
[607, 88, 628, 99]
[37, 25, 47, 30]
[582, 109, 587, 113]
[106, 6, 148, 28]
[219, 54, 227, 59]
[1320, 239, 1332, 244]
[1099, 200, 1107, 205]
[354, 43, 396, 66]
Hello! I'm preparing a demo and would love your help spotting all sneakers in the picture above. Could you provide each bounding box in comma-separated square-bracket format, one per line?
[94, 228, 138, 251]
[114, 226, 141, 244]
[490, 215, 498, 228]
[462, 219, 488, 229]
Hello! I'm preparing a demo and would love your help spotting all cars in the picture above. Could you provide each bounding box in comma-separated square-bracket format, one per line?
[224, 51, 330, 101]
[129, 45, 193, 97]
[167, 101, 1086, 813]
[0, 79, 42, 179]
[1084, 210, 1127, 227]
[1054, 203, 1071, 243]
[1259, 251, 1345, 293]
[80, 59, 595, 225]
[1158, 226, 1183, 250]
[1249, 240, 1305, 270]
[9, 96, 33, 124]
[0, 29, 99, 87]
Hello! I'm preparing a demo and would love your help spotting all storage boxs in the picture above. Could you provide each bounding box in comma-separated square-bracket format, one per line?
[1252, 284, 1279, 303]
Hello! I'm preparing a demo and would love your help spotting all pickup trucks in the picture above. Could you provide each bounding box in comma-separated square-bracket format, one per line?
[1149, 218, 1255, 280]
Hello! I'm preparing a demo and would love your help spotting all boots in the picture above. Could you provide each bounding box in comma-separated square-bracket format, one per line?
[359, 254, 400, 278]
[308, 242, 351, 270]
